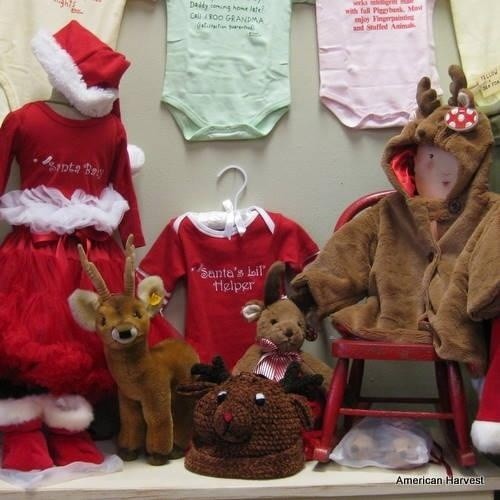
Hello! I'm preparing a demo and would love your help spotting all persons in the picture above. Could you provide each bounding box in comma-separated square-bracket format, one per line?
[0, 20, 184, 439]
[291, 65, 500, 379]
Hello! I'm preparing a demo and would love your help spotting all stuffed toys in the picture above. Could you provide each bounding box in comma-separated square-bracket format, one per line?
[68, 233, 200, 465]
[231, 260, 335, 396]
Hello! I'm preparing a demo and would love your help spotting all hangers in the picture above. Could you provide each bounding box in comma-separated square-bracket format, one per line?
[217, 163, 249, 213]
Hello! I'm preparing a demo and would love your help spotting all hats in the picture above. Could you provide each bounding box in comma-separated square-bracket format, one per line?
[176, 357, 325, 480]
[31, 20, 145, 173]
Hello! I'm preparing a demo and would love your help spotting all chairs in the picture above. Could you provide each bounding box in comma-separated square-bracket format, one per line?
[314, 188, 476, 469]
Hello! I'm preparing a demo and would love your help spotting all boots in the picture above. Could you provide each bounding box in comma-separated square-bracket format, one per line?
[0, 395, 53, 469]
[40, 394, 106, 465]
